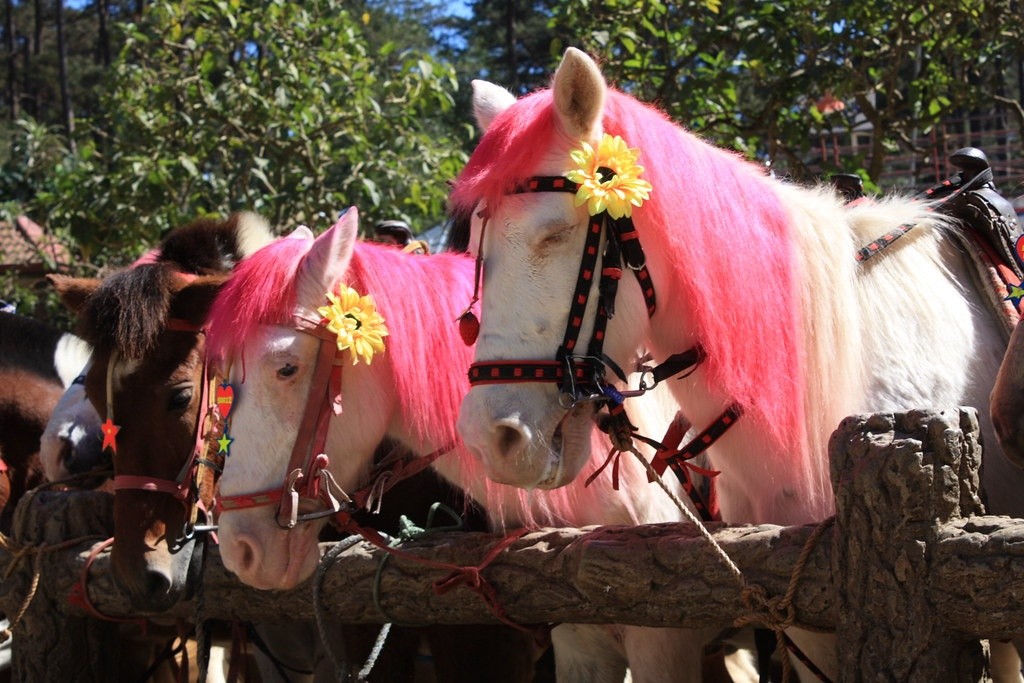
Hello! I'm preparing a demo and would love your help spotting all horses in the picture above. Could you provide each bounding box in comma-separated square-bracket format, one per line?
[0, 47, 1018, 682]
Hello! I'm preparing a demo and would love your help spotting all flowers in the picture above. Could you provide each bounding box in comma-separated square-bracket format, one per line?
[567, 133, 654, 219]
[317, 283, 392, 365]
